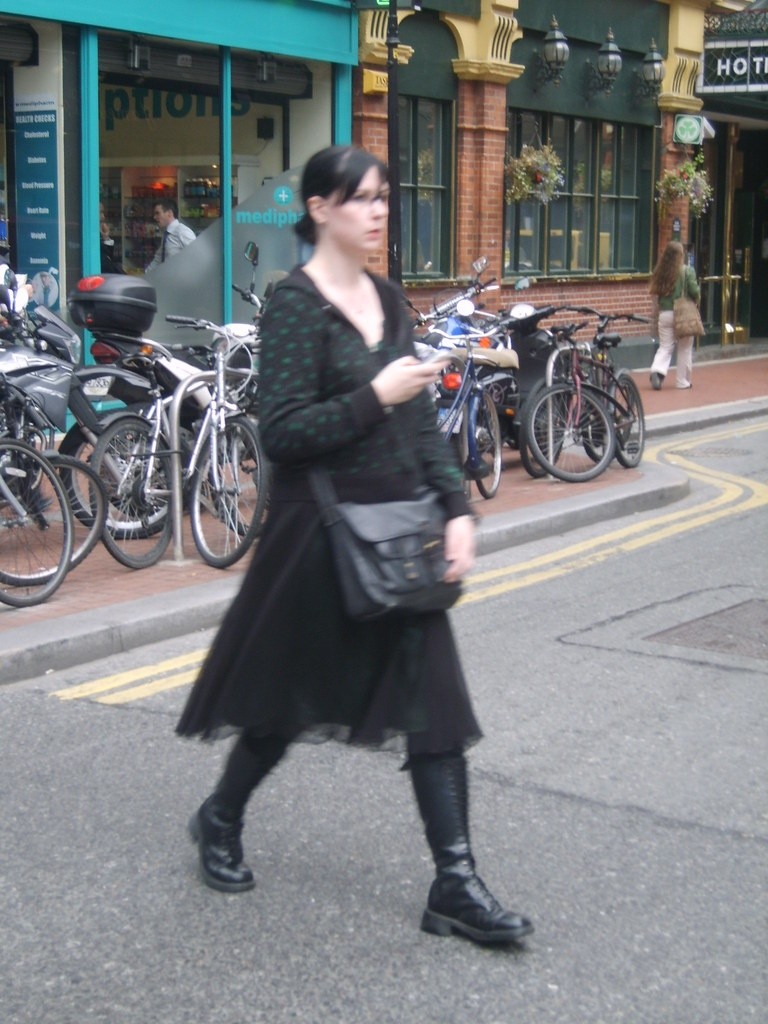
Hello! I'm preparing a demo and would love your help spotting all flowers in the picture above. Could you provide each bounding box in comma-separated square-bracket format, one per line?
[654, 147, 713, 224]
[502, 138, 567, 207]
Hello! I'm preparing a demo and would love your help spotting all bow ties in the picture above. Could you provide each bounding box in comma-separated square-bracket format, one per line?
[162, 232, 167, 263]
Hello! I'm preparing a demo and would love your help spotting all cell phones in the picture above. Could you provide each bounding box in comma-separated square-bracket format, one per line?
[423, 348, 451, 364]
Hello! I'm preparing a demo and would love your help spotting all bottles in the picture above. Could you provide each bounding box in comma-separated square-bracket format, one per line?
[182, 204, 221, 217]
[113, 240, 122, 261]
[128, 265, 145, 275]
[126, 243, 157, 255]
[132, 182, 177, 197]
[125, 220, 161, 237]
[99, 183, 122, 230]
[125, 203, 153, 216]
[184, 177, 221, 197]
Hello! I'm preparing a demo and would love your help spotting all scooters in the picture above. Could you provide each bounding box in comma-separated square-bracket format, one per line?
[435, 302, 568, 481]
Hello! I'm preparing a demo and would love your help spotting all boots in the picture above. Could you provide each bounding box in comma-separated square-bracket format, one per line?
[188, 738, 285, 892]
[411, 757, 535, 940]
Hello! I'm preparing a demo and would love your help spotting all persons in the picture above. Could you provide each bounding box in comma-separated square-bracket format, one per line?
[144, 200, 196, 273]
[648, 241, 701, 390]
[99, 200, 126, 275]
[186, 144, 532, 943]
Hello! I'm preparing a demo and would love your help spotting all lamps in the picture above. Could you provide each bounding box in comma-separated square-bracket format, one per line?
[254, 52, 279, 85]
[585, 24, 622, 102]
[125, 33, 153, 71]
[533, 14, 570, 92]
[632, 36, 669, 103]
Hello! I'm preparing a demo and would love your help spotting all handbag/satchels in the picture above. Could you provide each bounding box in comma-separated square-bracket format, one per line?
[327, 486, 462, 616]
[673, 298, 706, 340]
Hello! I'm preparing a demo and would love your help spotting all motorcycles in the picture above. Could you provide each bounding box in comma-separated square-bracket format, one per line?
[58, 241, 273, 538]
[405, 257, 500, 350]
[0, 265, 178, 538]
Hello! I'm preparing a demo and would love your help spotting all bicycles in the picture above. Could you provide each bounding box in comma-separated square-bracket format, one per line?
[0, 304, 651, 608]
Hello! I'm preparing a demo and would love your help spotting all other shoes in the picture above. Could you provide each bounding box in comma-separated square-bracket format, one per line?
[651, 373, 661, 390]
[686, 384, 692, 388]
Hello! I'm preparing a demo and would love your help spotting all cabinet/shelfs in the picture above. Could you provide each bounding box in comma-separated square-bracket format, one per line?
[99, 154, 262, 275]
